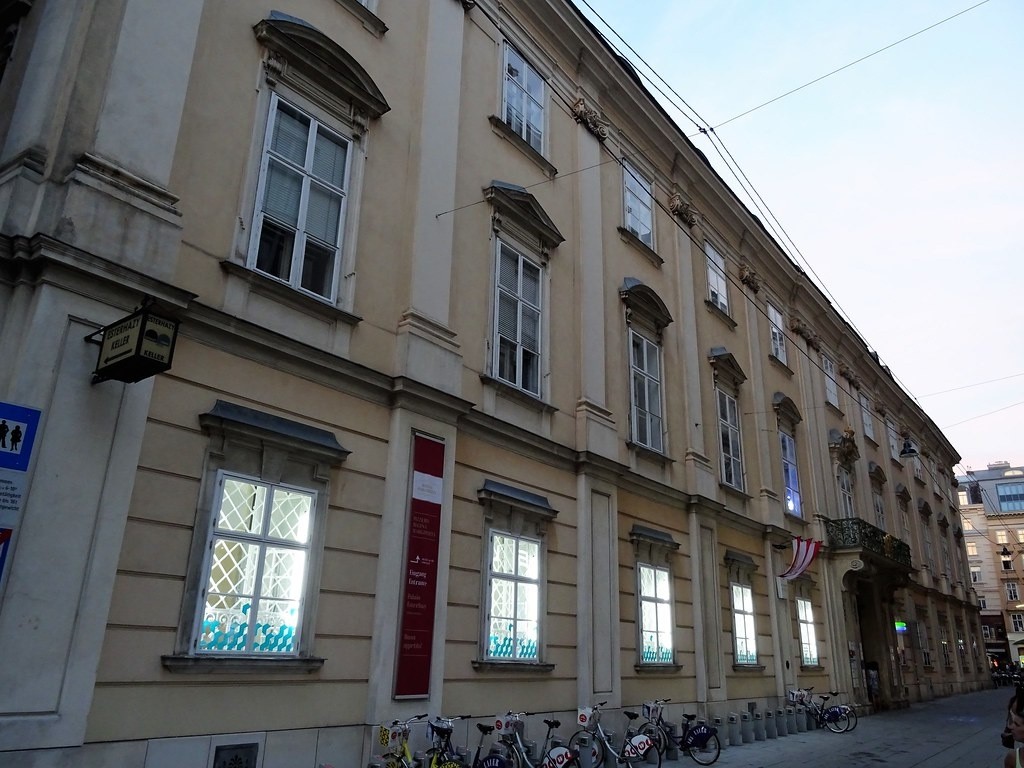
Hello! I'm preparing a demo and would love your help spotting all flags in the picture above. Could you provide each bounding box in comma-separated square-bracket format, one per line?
[776, 536, 824, 580]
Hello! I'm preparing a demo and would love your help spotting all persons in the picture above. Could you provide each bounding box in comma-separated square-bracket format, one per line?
[1004, 686, 1024, 768]
[991, 661, 1024, 686]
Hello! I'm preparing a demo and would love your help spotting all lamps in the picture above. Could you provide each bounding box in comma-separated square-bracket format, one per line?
[899, 441, 919, 458]
[1001, 544, 1012, 556]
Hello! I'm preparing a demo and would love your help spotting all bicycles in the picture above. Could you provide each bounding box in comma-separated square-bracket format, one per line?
[365, 713, 469, 768]
[425, 714, 515, 768]
[489, 709, 581, 768]
[790, 686, 858, 733]
[638, 697, 721, 766]
[567, 701, 661, 768]
